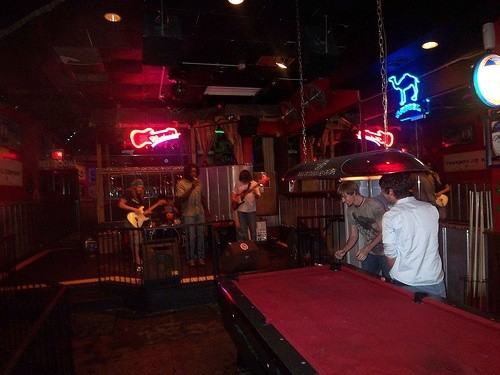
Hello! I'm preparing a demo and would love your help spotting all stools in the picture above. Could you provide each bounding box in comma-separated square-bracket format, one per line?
[141, 238, 179, 278]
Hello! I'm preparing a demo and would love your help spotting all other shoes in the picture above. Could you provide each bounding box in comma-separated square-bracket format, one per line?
[199, 259, 206, 266]
[190, 259, 196, 267]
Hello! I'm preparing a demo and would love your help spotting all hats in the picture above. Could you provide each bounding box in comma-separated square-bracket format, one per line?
[130, 179, 144, 186]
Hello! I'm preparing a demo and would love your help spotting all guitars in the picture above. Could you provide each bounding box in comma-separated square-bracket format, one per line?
[231, 177, 270, 210]
[127, 199, 166, 228]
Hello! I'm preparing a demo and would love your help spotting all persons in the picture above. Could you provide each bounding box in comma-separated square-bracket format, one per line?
[118, 179, 144, 265]
[177, 162, 210, 264]
[379, 161, 447, 300]
[426, 161, 450, 221]
[232, 170, 261, 242]
[334, 180, 391, 281]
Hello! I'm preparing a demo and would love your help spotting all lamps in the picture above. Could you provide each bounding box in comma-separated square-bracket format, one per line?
[280, 0, 431, 182]
[214, 124, 225, 134]
[276, 55, 289, 69]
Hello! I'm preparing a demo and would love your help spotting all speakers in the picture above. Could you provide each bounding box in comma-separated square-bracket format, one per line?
[142, 237, 182, 283]
[219, 239, 259, 272]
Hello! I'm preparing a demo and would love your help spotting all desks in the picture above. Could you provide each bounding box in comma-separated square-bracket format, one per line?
[213, 260, 500, 375]
